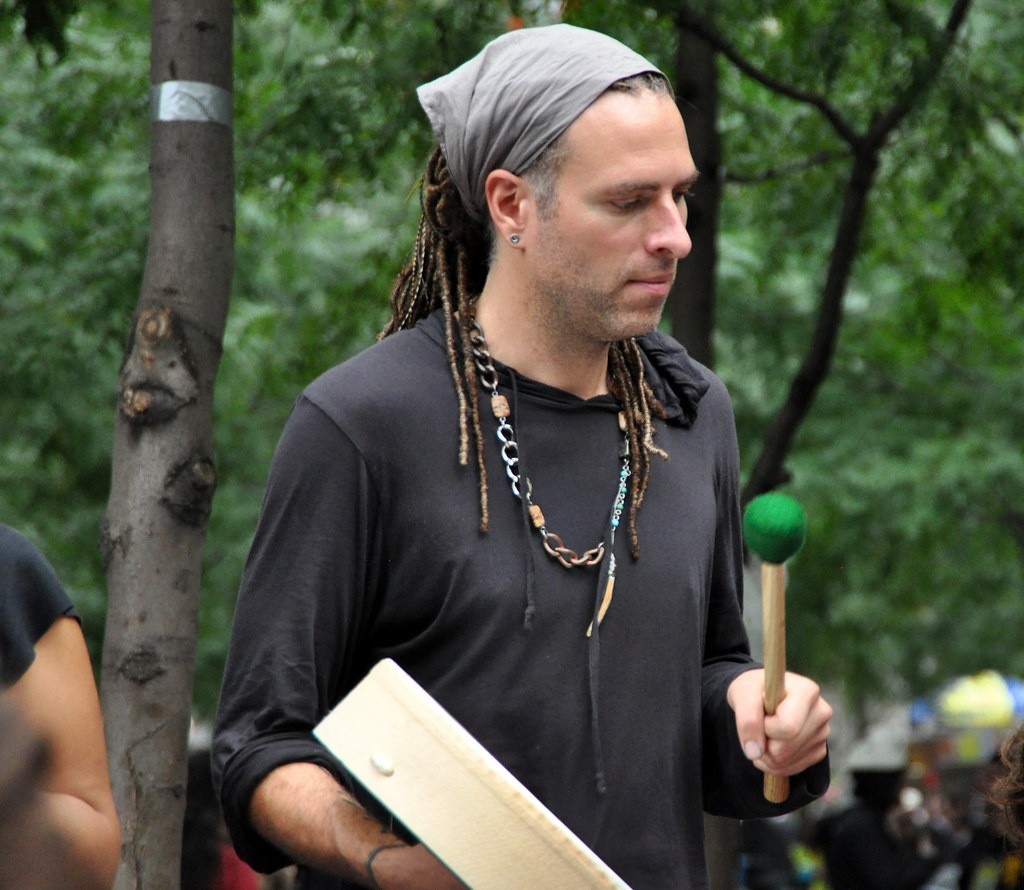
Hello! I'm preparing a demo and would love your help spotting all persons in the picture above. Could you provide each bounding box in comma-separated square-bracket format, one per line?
[209, 26, 832, 890]
[0, 521, 121, 890]
[787, 766, 1024, 889]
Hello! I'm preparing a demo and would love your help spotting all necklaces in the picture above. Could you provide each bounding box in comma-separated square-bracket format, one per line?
[465, 293, 632, 569]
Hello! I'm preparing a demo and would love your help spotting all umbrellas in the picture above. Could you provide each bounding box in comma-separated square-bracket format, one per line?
[912, 669, 1024, 730]
